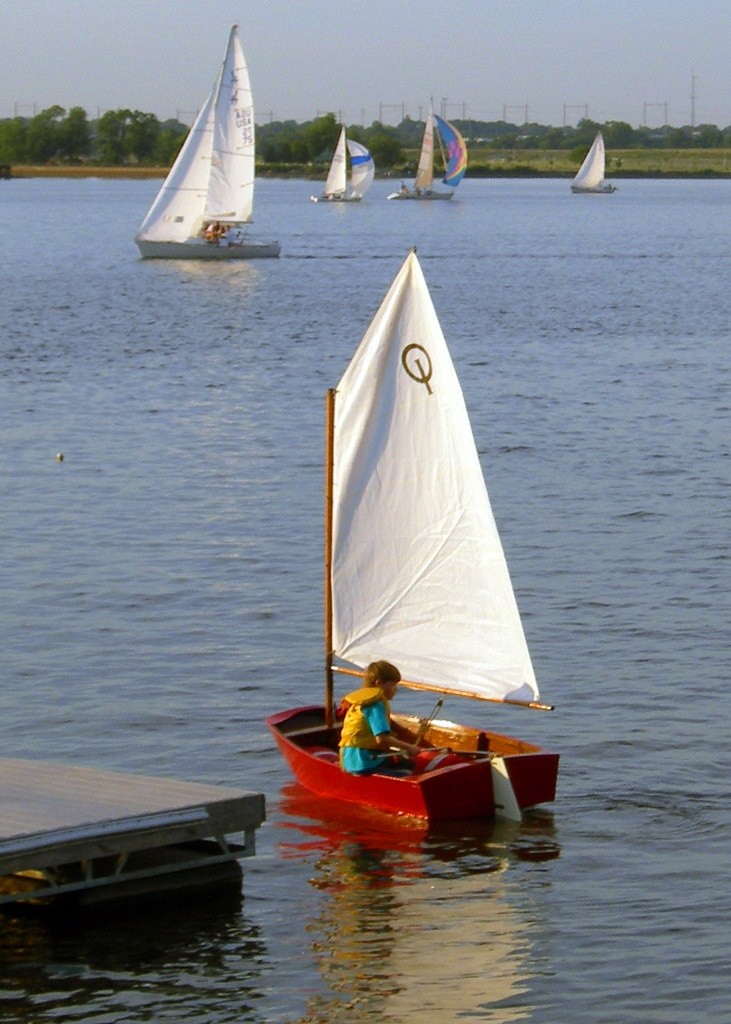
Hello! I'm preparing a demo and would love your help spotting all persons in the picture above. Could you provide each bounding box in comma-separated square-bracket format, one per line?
[207, 221, 233, 247]
[340, 660, 421, 776]
[400, 181, 420, 194]
[328, 192, 345, 200]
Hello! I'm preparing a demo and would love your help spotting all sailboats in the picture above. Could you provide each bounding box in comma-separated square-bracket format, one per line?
[388, 93, 469, 200]
[132, 23, 280, 261]
[310, 123, 375, 202]
[570, 125, 617, 194]
[264, 245, 559, 826]
